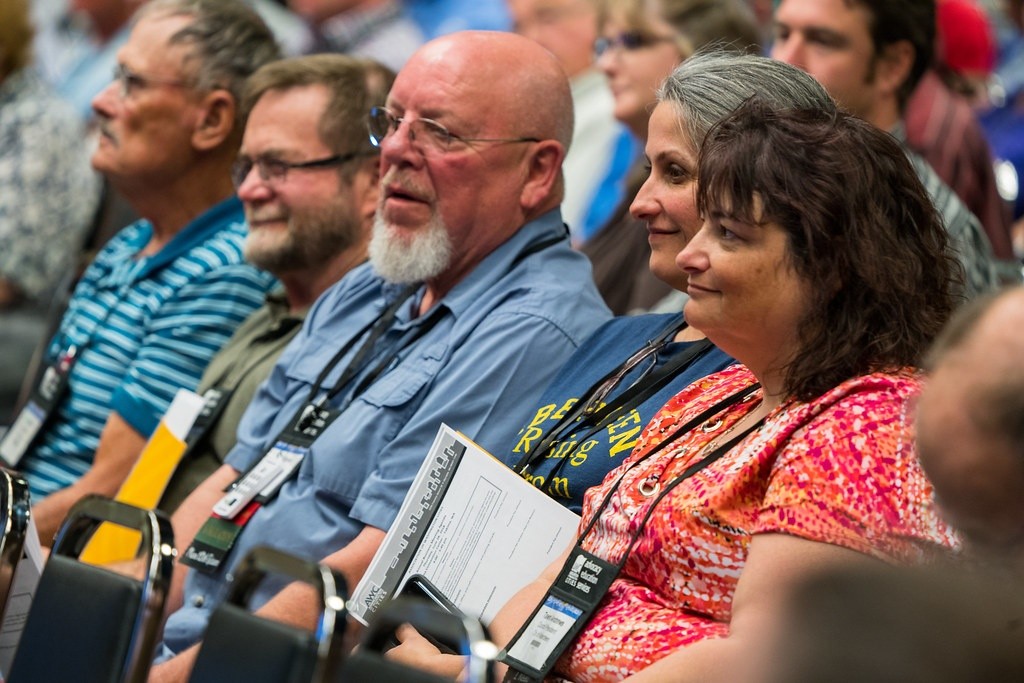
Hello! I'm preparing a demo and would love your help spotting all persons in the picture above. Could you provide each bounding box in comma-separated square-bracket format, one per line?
[1, 0, 1024, 683]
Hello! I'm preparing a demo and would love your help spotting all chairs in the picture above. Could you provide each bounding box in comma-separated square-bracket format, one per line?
[340, 600, 490, 683]
[0, 467, 32, 630]
[184, 541, 348, 683]
[5, 493, 176, 683]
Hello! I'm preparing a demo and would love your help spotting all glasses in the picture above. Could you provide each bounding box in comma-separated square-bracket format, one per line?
[366, 105, 540, 160]
[228, 151, 356, 185]
[112, 64, 193, 100]
[592, 28, 672, 56]
